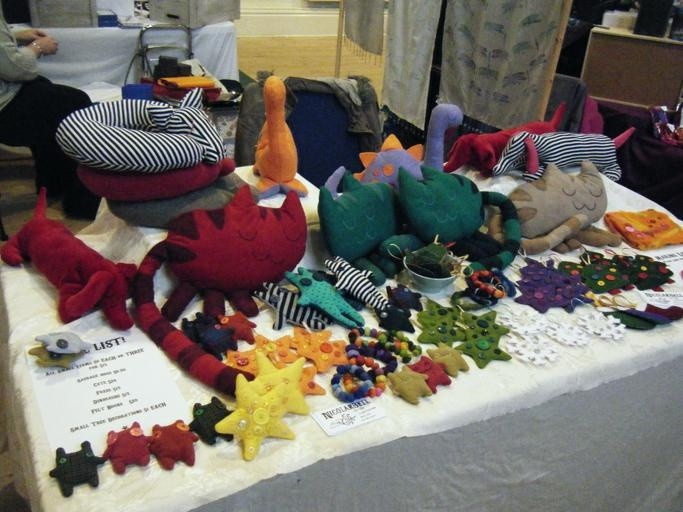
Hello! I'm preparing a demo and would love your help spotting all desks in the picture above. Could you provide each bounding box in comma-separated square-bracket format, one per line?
[1, 161, 683, 511]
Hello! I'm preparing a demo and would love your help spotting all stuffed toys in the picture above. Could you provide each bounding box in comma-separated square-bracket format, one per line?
[1, 75, 682, 499]
[3, 75, 635, 501]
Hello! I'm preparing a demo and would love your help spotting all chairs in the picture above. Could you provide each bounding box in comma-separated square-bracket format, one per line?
[237, 77, 384, 190]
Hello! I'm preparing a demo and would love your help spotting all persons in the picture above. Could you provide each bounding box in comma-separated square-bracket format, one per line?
[0, 1, 104, 221]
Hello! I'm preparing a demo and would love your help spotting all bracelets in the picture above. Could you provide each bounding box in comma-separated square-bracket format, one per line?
[31, 41, 43, 55]
